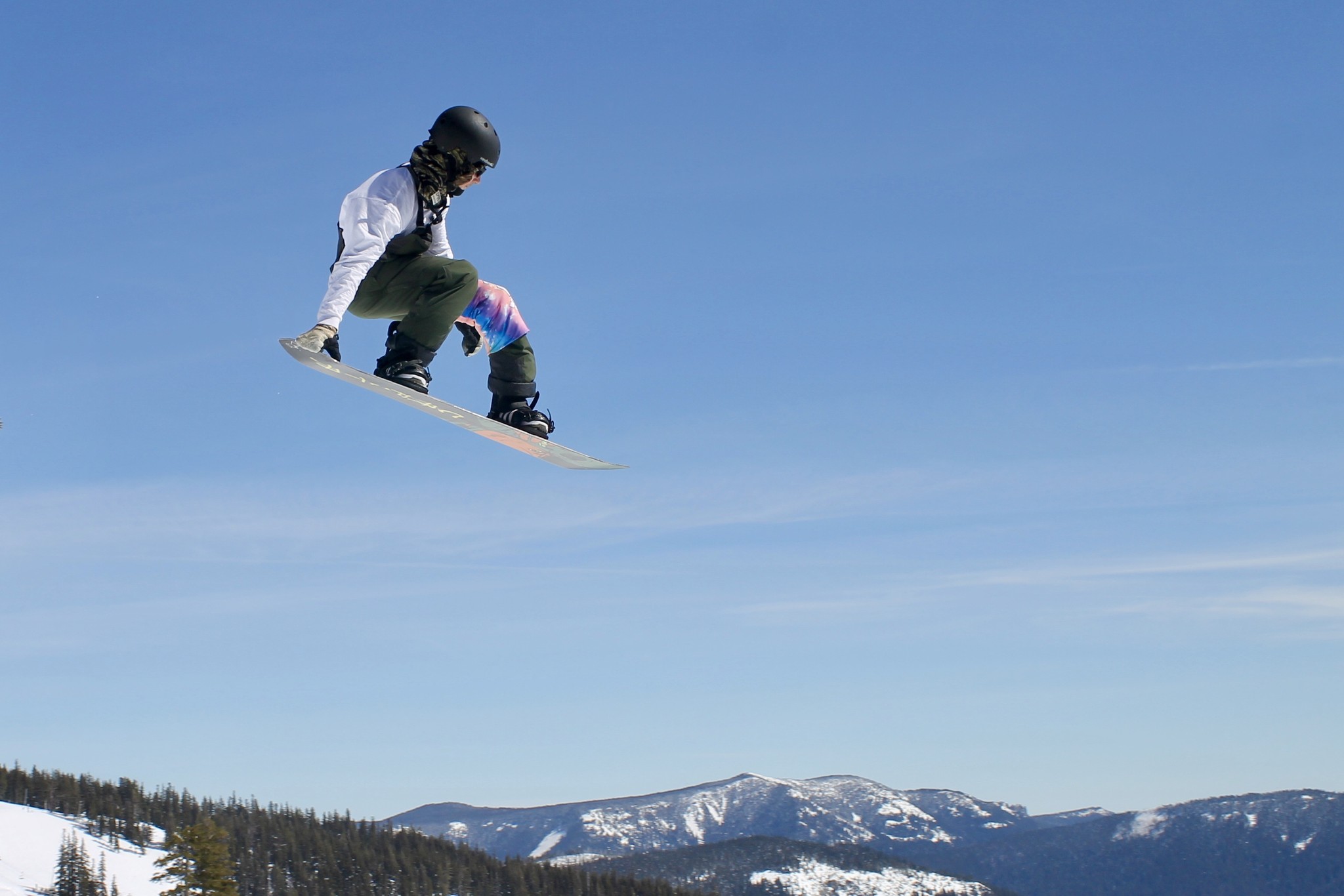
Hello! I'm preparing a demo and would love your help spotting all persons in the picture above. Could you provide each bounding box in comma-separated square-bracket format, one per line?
[293, 106, 555, 440]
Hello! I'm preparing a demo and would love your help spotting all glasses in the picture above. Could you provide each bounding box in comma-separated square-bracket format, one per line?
[473, 163, 486, 177]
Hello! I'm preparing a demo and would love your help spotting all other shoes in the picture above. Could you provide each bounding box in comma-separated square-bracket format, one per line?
[487, 393, 555, 440]
[374, 349, 432, 394]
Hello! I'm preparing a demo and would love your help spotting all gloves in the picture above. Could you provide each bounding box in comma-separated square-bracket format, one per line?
[296, 323, 341, 362]
[454, 321, 483, 357]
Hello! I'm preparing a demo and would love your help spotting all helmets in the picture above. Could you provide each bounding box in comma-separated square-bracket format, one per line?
[428, 106, 500, 168]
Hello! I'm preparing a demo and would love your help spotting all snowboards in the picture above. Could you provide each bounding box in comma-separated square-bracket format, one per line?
[276, 337, 632, 471]
